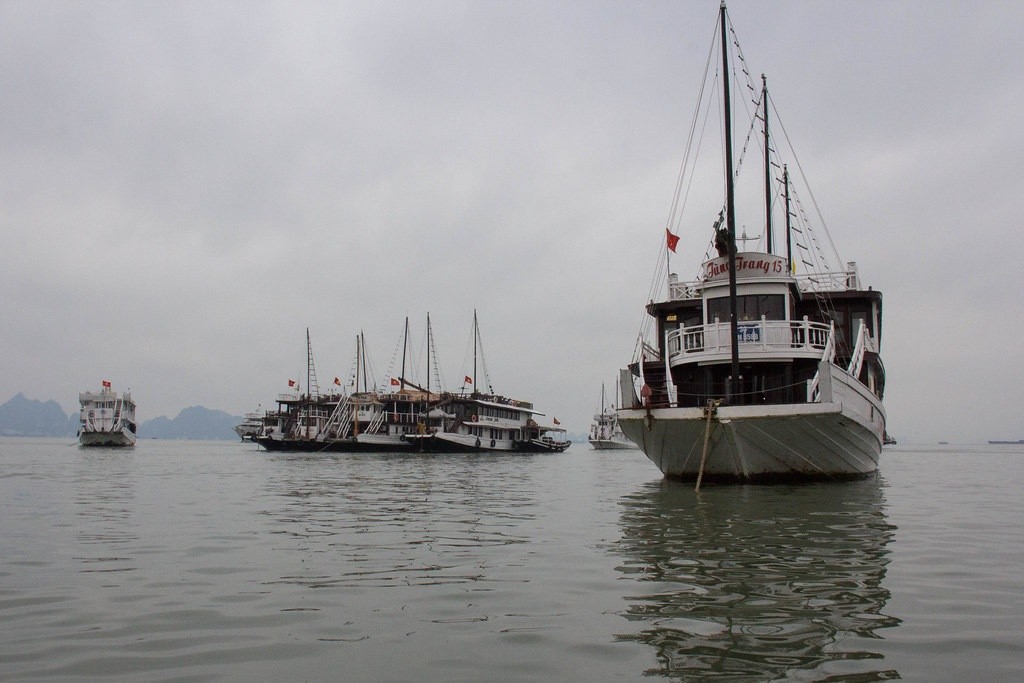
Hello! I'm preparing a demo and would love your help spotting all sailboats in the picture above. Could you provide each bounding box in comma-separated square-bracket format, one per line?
[232, 305, 546, 454]
[613, 0, 889, 487]
[588, 374, 641, 450]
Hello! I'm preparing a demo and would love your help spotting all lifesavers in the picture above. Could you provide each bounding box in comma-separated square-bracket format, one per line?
[567, 440, 571, 445]
[549, 445, 563, 451]
[491, 440, 496, 447]
[476, 440, 481, 446]
[471, 415, 476, 422]
[352, 437, 357, 443]
[511, 439, 533, 447]
[400, 435, 406, 442]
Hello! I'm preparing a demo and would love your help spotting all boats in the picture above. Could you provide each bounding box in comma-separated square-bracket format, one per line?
[76, 381, 140, 447]
[512, 424, 572, 454]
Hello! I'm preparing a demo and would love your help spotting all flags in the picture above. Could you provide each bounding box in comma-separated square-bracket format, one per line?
[391, 378, 399, 385]
[289, 380, 295, 386]
[103, 381, 111, 387]
[554, 417, 560, 425]
[667, 228, 680, 253]
[465, 376, 472, 384]
[334, 377, 340, 386]
